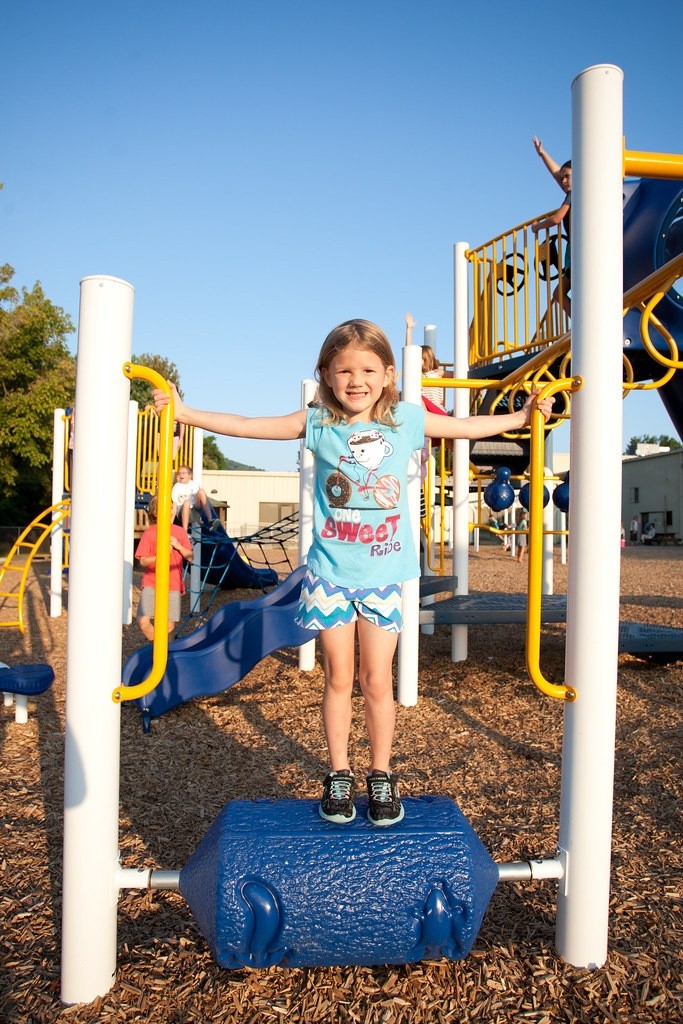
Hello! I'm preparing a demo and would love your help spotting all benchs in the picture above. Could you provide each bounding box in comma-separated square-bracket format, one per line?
[645, 538, 682, 545]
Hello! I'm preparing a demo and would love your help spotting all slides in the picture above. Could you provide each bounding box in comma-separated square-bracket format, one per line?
[120, 561, 318, 736]
[184, 499, 279, 588]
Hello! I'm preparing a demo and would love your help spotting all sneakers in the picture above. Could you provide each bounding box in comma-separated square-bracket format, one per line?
[319, 769, 357, 823]
[366, 769, 405, 826]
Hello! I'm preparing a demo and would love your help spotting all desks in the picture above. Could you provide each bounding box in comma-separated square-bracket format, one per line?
[654, 532, 679, 545]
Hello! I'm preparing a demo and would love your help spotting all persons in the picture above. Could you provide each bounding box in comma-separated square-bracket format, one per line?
[489, 515, 503, 541]
[172, 466, 221, 544]
[135, 495, 193, 642]
[641, 523, 655, 543]
[406, 313, 453, 416]
[630, 516, 638, 546]
[150, 318, 555, 826]
[621, 522, 626, 557]
[516, 512, 527, 563]
[533, 136, 572, 318]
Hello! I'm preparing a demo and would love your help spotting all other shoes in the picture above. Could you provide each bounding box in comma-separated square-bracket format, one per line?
[208, 518, 220, 532]
